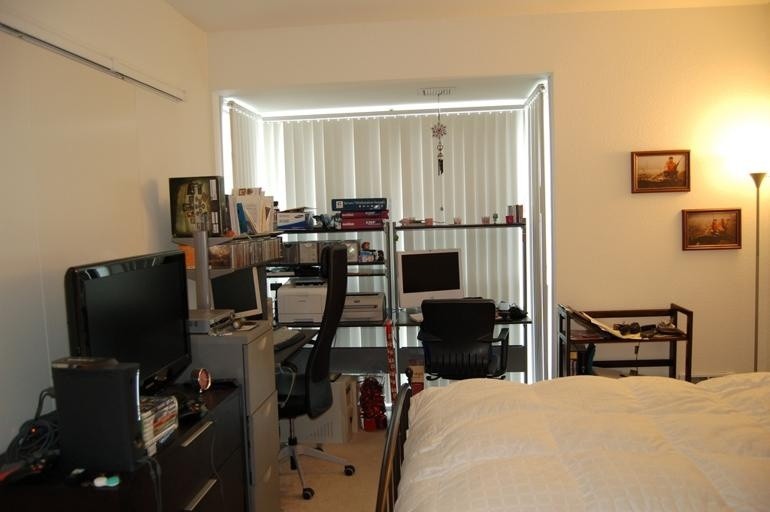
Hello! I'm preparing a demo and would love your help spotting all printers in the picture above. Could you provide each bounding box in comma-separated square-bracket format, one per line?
[277, 277, 328, 323]
[339, 292, 387, 321]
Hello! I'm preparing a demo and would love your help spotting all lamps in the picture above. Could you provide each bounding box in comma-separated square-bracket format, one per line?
[745, 146, 766, 372]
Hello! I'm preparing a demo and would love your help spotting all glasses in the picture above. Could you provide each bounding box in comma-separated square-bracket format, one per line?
[613, 322, 640, 336]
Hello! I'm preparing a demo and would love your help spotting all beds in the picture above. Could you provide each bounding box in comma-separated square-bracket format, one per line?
[376, 364, 769, 512]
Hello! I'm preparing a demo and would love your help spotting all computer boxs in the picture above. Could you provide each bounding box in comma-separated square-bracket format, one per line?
[51, 356, 146, 472]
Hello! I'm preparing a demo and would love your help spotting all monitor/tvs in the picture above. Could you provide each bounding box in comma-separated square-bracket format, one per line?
[63, 249, 193, 396]
[209, 267, 263, 318]
[395, 248, 464, 308]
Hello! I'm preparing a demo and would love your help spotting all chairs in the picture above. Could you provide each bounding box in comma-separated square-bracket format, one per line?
[270, 243, 356, 500]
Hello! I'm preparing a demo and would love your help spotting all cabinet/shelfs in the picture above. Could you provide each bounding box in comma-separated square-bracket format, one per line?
[177, 222, 537, 481]
[557, 303, 697, 382]
[0, 376, 253, 512]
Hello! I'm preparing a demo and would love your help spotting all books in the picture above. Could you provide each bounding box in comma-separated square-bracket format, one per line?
[140, 394, 179, 450]
[206, 187, 283, 271]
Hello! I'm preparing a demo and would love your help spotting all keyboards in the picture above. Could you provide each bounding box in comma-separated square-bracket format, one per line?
[409, 312, 424, 322]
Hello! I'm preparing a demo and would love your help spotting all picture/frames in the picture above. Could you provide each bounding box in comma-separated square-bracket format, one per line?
[681, 205, 743, 251]
[629, 146, 691, 195]
